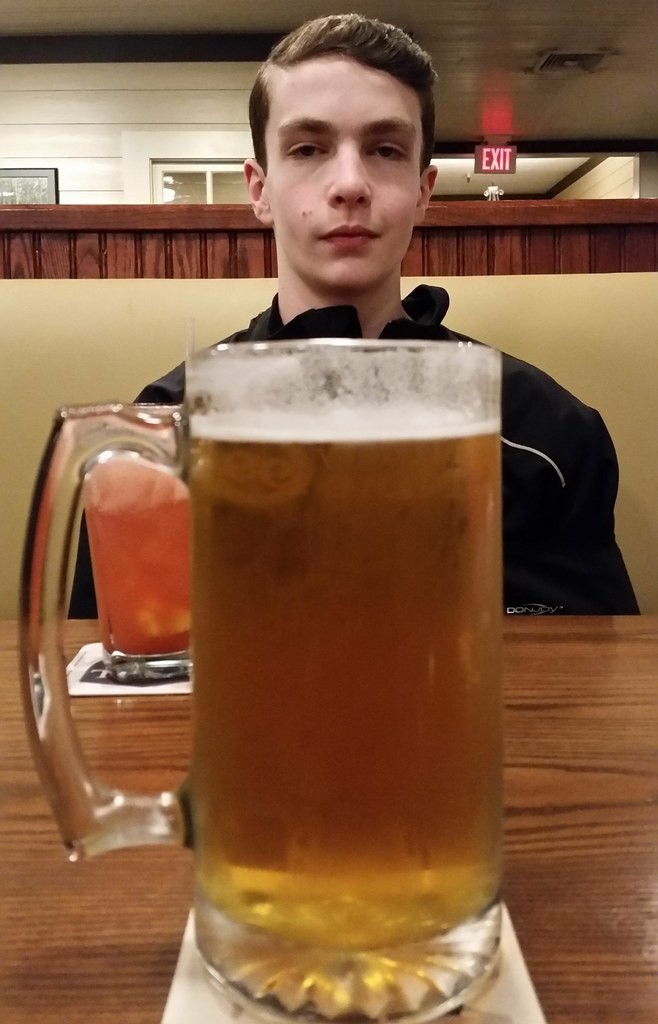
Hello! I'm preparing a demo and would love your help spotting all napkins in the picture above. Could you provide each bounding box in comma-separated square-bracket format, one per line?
[159, 900, 547, 1024]
[66, 642, 191, 696]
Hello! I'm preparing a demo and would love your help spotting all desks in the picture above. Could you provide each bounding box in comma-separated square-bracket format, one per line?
[0, 616, 658, 1024]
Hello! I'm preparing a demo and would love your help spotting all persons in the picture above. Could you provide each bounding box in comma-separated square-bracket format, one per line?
[67, 14, 640, 619]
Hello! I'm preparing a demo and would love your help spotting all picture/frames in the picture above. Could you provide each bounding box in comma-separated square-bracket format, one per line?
[0, 167, 59, 204]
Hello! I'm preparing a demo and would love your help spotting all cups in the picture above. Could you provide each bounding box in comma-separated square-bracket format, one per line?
[81, 404, 190, 676]
[19, 338, 503, 1024]
